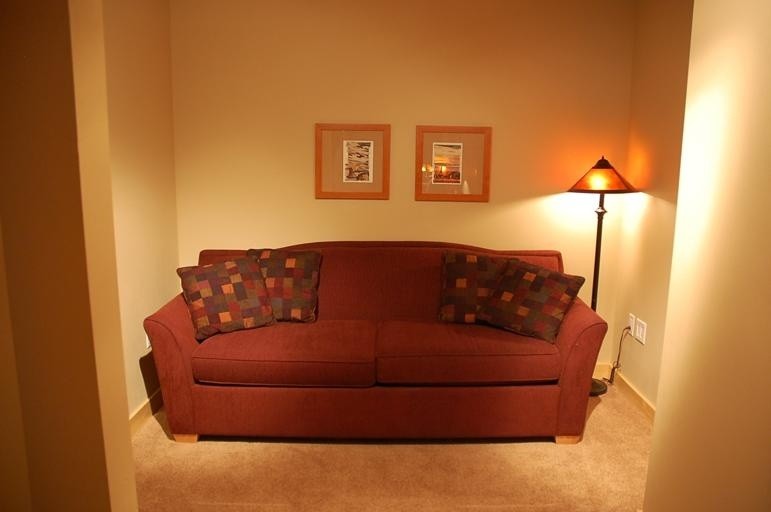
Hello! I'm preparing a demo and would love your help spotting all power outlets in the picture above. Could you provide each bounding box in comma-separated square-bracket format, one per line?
[627, 313, 635, 337]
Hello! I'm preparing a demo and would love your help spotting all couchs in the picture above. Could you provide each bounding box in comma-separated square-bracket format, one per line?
[143, 242, 607, 445]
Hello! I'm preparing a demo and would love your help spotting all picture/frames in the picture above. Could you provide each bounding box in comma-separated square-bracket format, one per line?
[415, 126, 492, 202]
[315, 123, 391, 200]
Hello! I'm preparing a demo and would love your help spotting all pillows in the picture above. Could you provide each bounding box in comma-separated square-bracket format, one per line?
[476, 258, 585, 344]
[177, 255, 278, 340]
[438, 250, 509, 325]
[247, 249, 321, 322]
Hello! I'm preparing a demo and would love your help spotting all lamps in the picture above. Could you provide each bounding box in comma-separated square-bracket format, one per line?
[566, 155, 640, 396]
